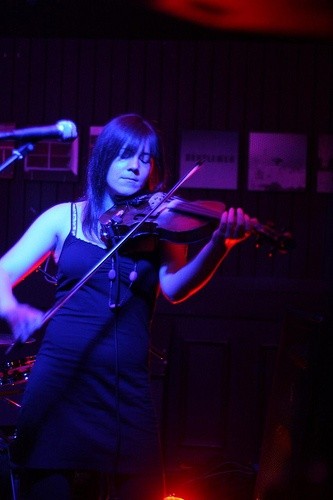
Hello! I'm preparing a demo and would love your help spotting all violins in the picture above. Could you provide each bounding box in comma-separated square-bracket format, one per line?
[99, 191, 293, 259]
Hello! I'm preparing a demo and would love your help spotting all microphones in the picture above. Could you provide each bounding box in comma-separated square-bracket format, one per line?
[0, 119, 78, 144]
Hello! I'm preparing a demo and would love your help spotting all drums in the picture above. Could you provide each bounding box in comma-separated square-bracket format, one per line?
[0, 356, 37, 426]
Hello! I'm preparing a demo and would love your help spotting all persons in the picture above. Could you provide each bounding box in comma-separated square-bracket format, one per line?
[0, 114, 259, 496]
